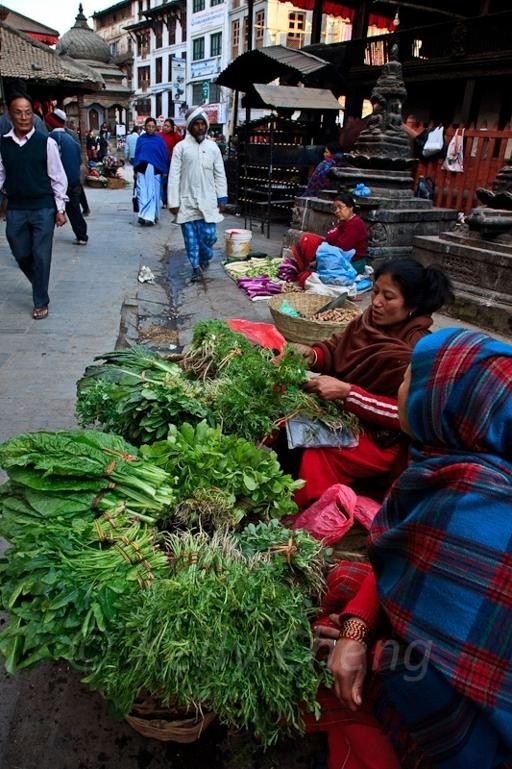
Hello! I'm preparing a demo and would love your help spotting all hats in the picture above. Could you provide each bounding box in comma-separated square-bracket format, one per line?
[44, 108, 67, 130]
[184, 106, 210, 131]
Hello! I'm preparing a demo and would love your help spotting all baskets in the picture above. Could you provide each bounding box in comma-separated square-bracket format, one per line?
[102, 688, 217, 745]
[266, 291, 363, 348]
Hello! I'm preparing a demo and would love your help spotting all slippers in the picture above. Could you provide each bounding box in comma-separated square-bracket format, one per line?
[33, 307, 48, 319]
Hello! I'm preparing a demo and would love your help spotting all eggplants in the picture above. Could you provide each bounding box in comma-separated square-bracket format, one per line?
[234, 276, 283, 299]
[277, 257, 298, 281]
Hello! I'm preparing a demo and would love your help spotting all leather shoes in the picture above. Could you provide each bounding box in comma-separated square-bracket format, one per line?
[190, 267, 202, 283]
[201, 259, 210, 270]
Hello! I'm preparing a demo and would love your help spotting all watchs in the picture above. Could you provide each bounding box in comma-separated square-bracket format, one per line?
[57, 210, 65, 214]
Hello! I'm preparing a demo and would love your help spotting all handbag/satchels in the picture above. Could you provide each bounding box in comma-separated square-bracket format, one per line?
[132, 178, 140, 214]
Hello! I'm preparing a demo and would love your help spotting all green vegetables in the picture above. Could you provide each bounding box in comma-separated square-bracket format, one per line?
[0, 317, 362, 749]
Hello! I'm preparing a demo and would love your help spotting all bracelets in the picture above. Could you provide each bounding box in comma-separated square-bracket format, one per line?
[310, 348, 318, 368]
[338, 620, 370, 649]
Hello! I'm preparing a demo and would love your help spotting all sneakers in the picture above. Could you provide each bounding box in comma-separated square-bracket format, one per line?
[71, 238, 88, 246]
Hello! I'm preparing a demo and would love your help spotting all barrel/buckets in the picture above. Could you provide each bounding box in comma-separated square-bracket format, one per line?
[223, 228, 252, 258]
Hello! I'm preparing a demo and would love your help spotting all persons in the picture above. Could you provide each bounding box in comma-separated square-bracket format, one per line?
[125, 126, 140, 165]
[166, 107, 228, 283]
[102, 155, 118, 176]
[100, 121, 109, 139]
[86, 127, 98, 150]
[305, 144, 343, 195]
[0, 109, 51, 219]
[0, 94, 69, 320]
[158, 119, 183, 162]
[310, 326, 512, 769]
[292, 194, 369, 284]
[133, 117, 170, 226]
[42, 108, 89, 246]
[95, 135, 108, 161]
[64, 126, 90, 217]
[275, 259, 451, 512]
